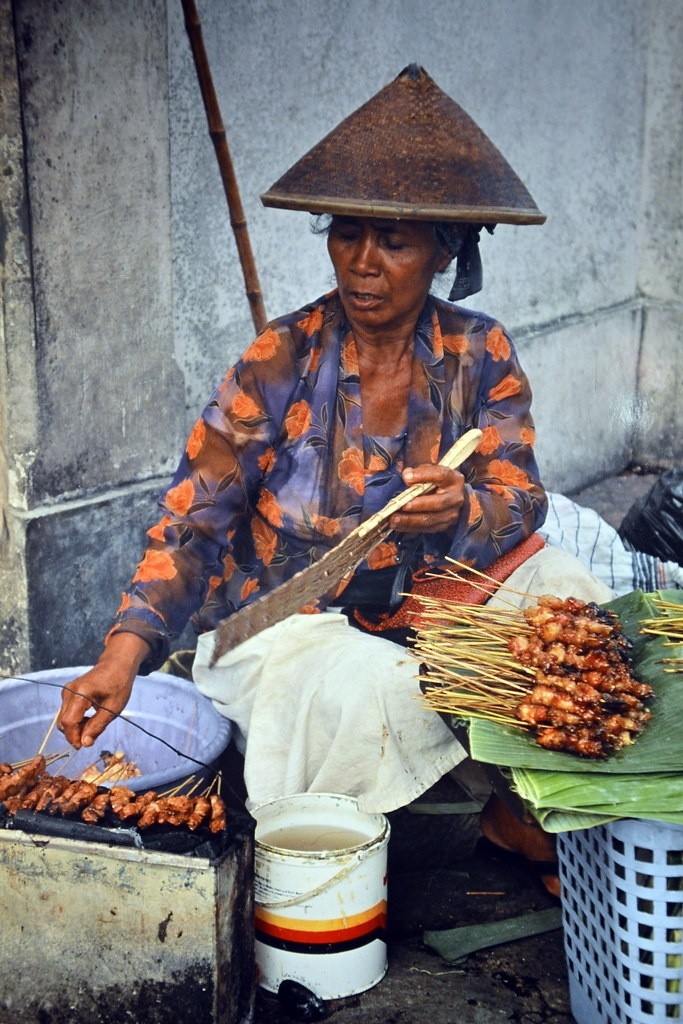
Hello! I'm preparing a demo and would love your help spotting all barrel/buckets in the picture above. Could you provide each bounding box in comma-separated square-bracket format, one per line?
[249, 791, 389, 1000]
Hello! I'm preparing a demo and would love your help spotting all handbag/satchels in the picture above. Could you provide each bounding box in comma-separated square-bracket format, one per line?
[353, 532, 545, 636]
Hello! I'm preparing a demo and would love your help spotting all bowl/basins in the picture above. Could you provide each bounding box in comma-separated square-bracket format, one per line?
[0, 665, 229, 799]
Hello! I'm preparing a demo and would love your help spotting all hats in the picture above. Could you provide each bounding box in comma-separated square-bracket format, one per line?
[259, 63, 547, 226]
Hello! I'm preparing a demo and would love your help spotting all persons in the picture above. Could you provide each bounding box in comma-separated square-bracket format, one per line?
[55, 66, 614, 913]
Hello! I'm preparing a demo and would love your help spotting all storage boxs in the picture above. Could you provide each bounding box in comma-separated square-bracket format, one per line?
[557, 816, 683, 1024]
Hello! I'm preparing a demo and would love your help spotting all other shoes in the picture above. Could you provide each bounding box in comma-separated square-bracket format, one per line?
[472, 787, 580, 907]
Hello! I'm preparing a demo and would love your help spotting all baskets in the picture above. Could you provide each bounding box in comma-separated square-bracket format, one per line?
[555, 820, 683, 1024]
